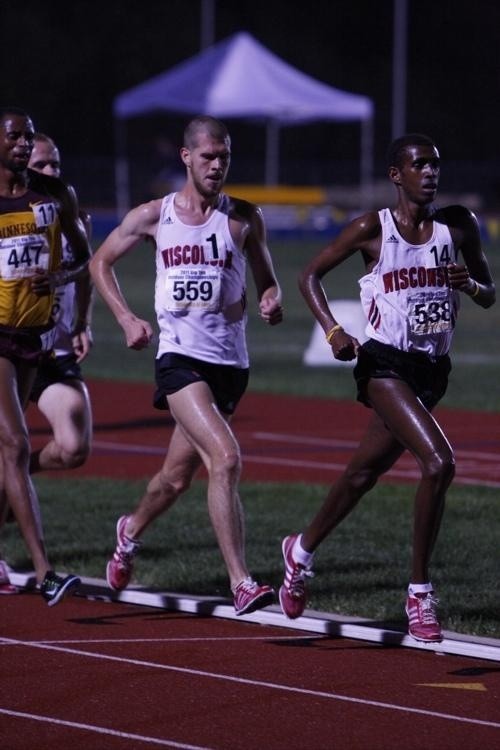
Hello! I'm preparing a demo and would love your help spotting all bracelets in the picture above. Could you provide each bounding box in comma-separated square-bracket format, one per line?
[325, 324, 342, 343]
[472, 282, 480, 298]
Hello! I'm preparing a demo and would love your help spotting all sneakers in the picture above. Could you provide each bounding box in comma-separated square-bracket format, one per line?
[279, 533, 314, 619]
[404, 590, 443, 643]
[1, 560, 20, 595]
[233, 579, 275, 616]
[105, 514, 144, 592]
[41, 570, 81, 607]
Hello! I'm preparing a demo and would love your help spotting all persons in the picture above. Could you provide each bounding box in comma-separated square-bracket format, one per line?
[0, 131, 92, 593]
[0, 107, 100, 610]
[88, 115, 284, 616]
[277, 131, 499, 642]
